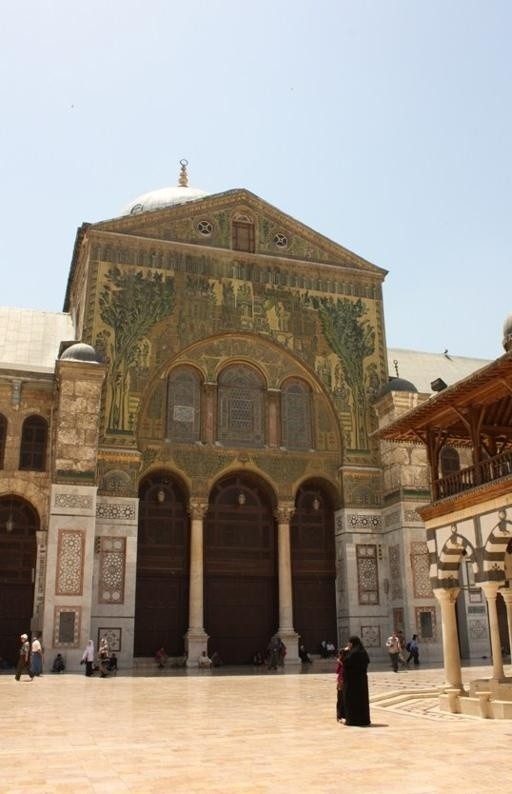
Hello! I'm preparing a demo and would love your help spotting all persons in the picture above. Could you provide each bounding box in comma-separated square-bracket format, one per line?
[80, 639, 118, 678]
[53, 653, 65, 674]
[253, 636, 336, 670]
[386, 631, 419, 673]
[15, 633, 35, 681]
[31, 632, 42, 677]
[339, 636, 370, 726]
[336, 648, 345, 722]
[155, 646, 223, 669]
